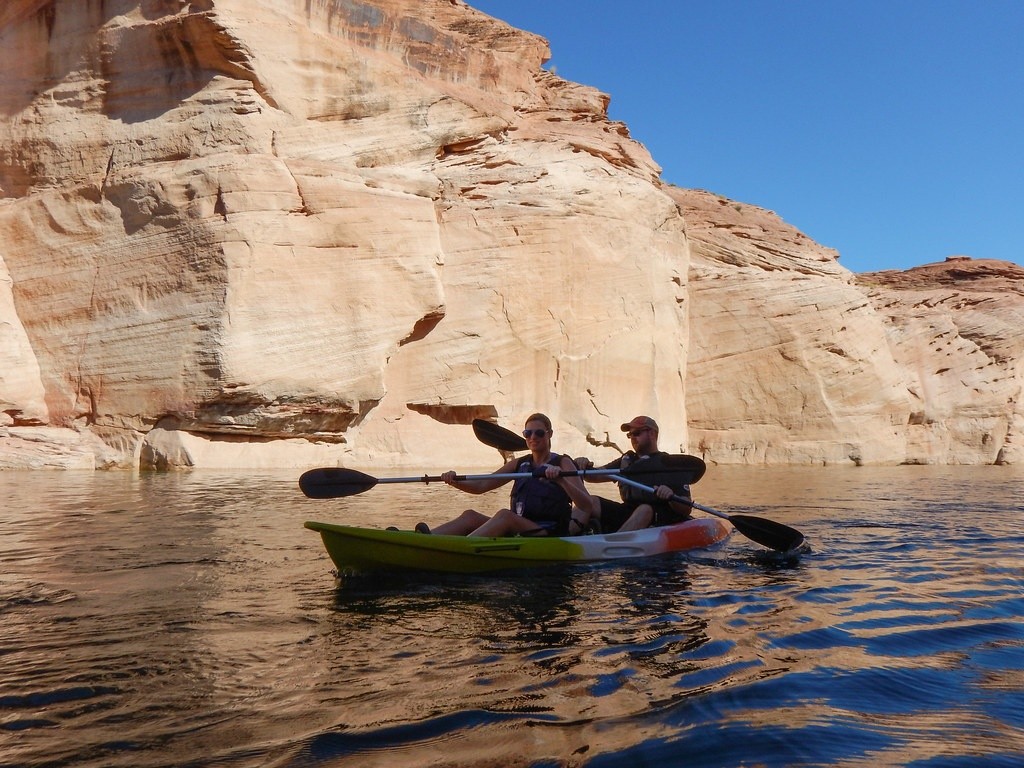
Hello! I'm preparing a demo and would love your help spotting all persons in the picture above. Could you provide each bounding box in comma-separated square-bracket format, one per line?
[570, 416, 692, 536]
[386, 413, 593, 537]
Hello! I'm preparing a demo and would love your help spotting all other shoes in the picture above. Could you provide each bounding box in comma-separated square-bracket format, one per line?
[415, 523, 432, 535]
[387, 527, 400, 532]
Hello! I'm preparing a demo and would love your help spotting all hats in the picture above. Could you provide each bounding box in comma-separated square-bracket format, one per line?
[621, 415, 658, 433]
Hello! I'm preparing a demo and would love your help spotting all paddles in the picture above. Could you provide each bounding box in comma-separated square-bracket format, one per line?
[297, 453, 708, 500]
[471, 418, 806, 553]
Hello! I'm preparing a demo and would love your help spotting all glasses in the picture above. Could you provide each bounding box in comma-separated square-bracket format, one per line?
[522, 430, 548, 438]
[627, 429, 647, 438]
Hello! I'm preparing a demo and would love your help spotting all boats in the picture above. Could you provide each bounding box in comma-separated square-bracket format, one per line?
[301, 514, 734, 574]
